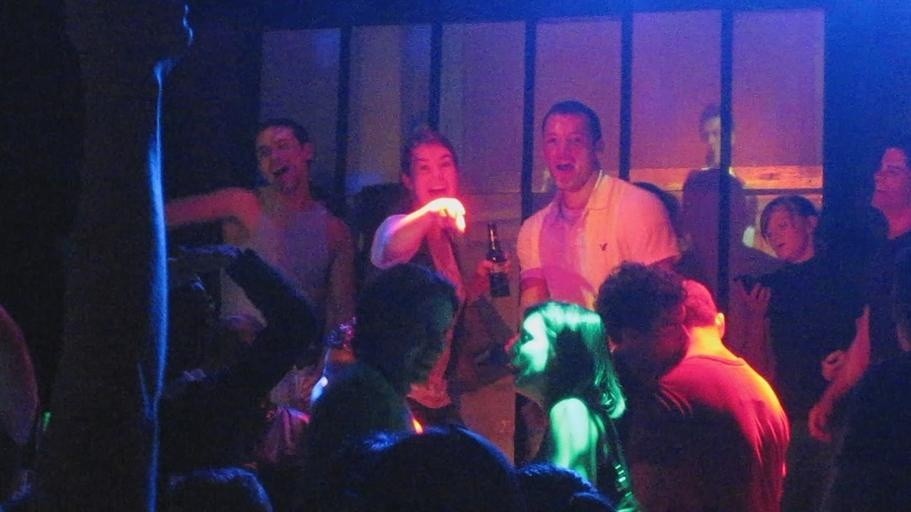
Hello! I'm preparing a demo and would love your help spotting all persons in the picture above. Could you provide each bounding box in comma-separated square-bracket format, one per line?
[801, 138, 911, 512]
[367, 118, 519, 425]
[162, 116, 362, 399]
[511, 95, 684, 326]
[3, 194, 911, 512]
[2, 0, 199, 512]
[670, 103, 737, 191]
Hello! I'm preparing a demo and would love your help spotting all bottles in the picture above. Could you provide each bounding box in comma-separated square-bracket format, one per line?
[484, 218, 513, 299]
